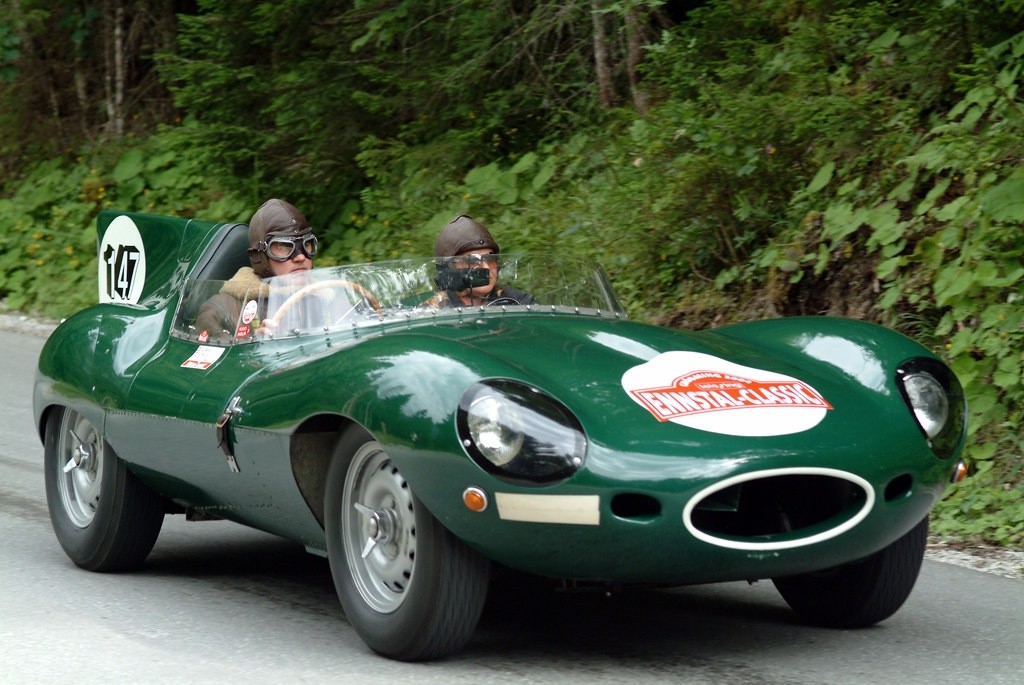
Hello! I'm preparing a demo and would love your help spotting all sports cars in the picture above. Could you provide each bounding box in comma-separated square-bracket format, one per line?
[30, 210, 970, 665]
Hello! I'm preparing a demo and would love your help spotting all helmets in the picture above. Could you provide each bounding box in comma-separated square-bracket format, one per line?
[436, 214, 499, 270]
[248, 198, 313, 279]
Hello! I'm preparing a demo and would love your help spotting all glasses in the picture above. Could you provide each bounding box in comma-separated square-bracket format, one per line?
[265, 234, 319, 263]
[447, 252, 498, 266]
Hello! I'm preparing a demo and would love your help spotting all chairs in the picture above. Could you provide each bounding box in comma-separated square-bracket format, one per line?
[184, 221, 252, 324]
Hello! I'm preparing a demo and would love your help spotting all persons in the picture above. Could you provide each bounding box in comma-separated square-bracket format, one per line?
[196, 198, 366, 338]
[417, 215, 535, 308]
